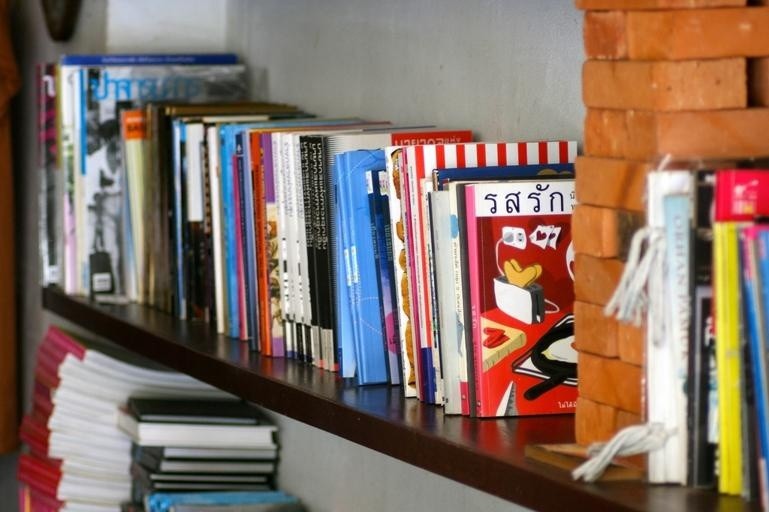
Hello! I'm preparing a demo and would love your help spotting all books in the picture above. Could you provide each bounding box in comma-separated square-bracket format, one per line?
[12, 50, 575, 511]
[644, 160, 769, 511]
